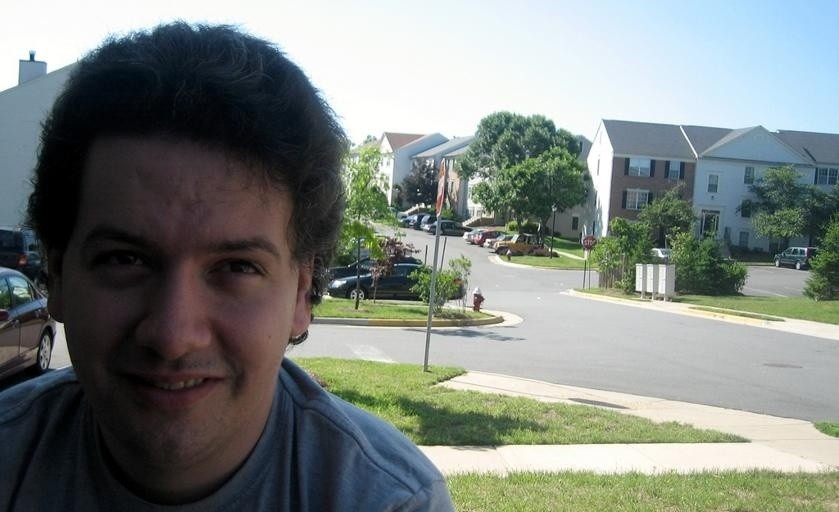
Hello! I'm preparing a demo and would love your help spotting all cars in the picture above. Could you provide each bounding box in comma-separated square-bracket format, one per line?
[0, 267, 56, 380]
[652, 248, 668, 262]
[774, 247, 818, 270]
[397, 206, 472, 235]
[0, 230, 40, 267]
[463, 229, 548, 256]
[327, 257, 465, 300]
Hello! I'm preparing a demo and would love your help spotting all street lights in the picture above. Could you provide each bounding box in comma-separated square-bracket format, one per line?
[551, 204, 557, 258]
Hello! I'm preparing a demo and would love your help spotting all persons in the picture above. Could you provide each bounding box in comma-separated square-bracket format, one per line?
[1, 21, 454, 511]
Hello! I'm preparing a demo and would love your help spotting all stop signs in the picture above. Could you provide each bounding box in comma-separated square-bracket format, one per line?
[583, 235, 595, 249]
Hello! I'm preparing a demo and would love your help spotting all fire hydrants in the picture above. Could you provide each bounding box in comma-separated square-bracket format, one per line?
[473, 288, 484, 311]
[506, 249, 512, 260]
